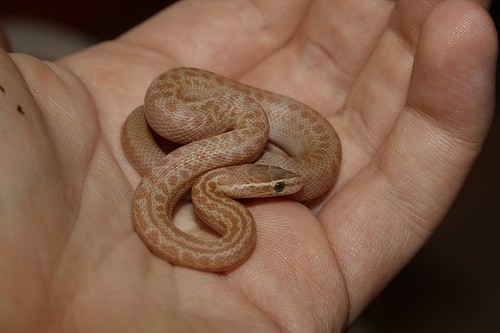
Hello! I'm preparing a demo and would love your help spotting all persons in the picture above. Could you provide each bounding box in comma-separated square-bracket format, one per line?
[0, 0, 498, 332]
